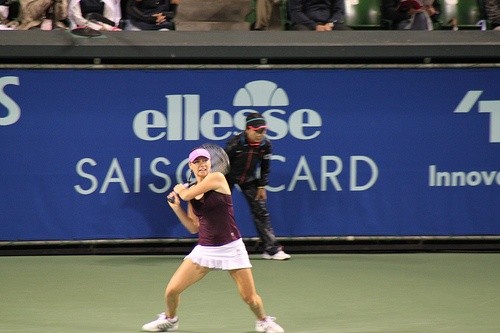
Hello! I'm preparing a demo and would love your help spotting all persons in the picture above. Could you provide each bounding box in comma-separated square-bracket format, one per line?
[223, 113, 291, 261]
[1, 0, 500, 32]
[142, 147, 284, 333]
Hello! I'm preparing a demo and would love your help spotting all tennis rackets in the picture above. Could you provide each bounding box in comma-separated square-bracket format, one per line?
[168, 143, 231, 203]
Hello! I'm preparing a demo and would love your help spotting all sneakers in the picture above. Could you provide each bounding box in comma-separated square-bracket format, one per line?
[263, 250, 291, 259]
[256, 316, 284, 333]
[142, 312, 179, 331]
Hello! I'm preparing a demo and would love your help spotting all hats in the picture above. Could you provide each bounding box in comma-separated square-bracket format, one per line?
[189, 149, 210, 162]
[247, 118, 267, 130]
